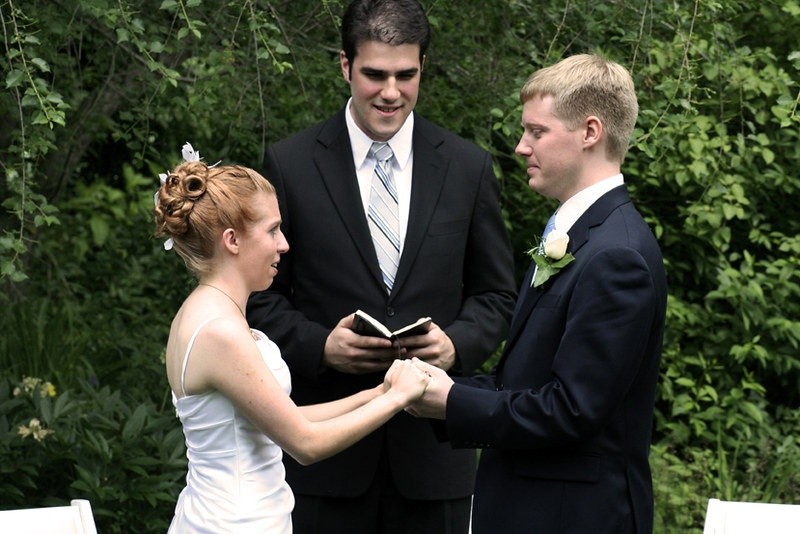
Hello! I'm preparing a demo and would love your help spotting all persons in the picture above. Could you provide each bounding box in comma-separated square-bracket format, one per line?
[247, 0, 517, 534]
[154, 159, 430, 534]
[382, 53, 664, 534]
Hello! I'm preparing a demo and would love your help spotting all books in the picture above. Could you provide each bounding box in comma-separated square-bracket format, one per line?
[351, 311, 432, 351]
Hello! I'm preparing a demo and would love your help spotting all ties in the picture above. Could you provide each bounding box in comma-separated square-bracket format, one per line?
[366, 142, 400, 295]
[530, 214, 557, 286]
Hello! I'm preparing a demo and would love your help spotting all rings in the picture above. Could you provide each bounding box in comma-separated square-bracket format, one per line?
[425, 370, 429, 376]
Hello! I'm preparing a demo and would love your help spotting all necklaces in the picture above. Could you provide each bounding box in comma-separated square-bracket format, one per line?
[200, 284, 245, 316]
